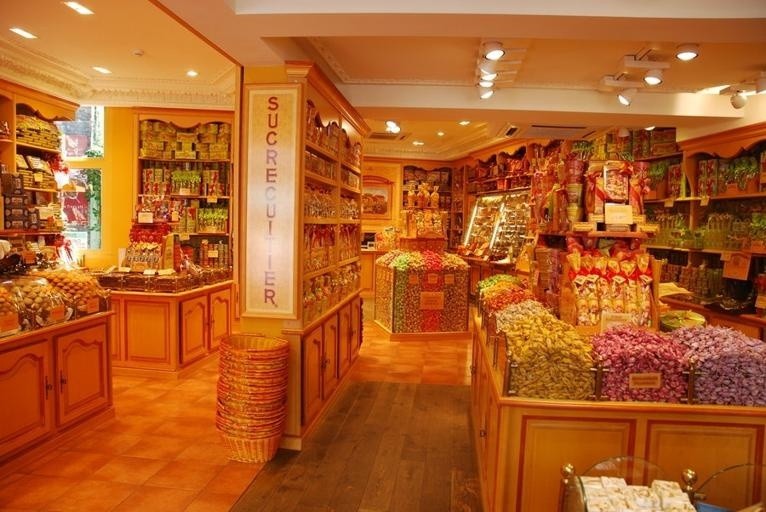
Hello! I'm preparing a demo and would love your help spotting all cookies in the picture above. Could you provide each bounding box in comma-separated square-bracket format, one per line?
[0, 270, 107, 314]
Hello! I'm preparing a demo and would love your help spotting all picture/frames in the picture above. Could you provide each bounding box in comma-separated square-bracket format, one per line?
[233, 79, 303, 325]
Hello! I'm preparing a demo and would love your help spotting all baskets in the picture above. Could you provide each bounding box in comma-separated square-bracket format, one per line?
[216, 335, 288, 463]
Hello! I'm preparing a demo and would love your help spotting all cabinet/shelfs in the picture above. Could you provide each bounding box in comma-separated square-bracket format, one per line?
[372, 243, 472, 337]
[555, 221, 665, 334]
[286, 291, 363, 451]
[471, 301, 765, 510]
[467, 260, 515, 298]
[361, 156, 452, 297]
[598, 123, 766, 339]
[455, 132, 594, 260]
[0, 80, 81, 239]
[134, 107, 237, 266]
[108, 281, 238, 380]
[0, 305, 118, 463]
[299, 101, 360, 313]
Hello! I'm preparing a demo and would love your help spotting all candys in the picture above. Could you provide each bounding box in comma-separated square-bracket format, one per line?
[376, 250, 468, 334]
[476, 272, 766, 404]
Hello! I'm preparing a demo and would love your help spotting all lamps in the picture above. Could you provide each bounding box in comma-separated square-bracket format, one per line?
[477, 44, 506, 99]
[607, 41, 761, 109]
